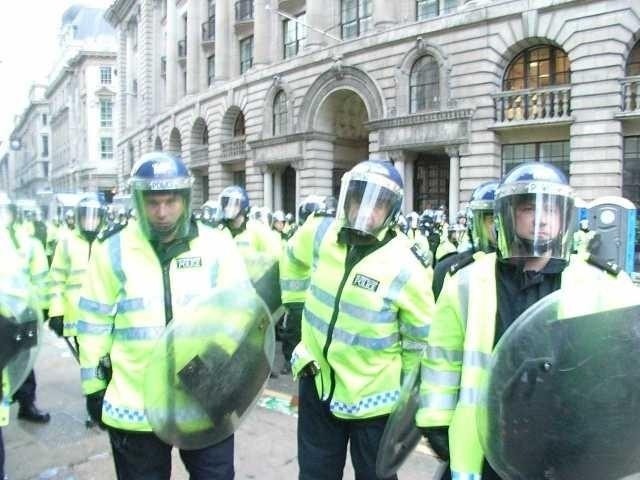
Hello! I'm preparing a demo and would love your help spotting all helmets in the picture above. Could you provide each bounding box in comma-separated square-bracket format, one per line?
[131, 153, 194, 239]
[195, 184, 295, 233]
[336, 159, 403, 240]
[298, 196, 322, 223]
[466, 182, 494, 253]
[494, 162, 575, 262]
[405, 208, 466, 244]
[0, 189, 127, 233]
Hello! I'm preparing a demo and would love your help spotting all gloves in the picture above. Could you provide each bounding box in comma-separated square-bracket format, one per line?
[422, 427, 451, 461]
[87, 394, 107, 429]
[49, 316, 63, 337]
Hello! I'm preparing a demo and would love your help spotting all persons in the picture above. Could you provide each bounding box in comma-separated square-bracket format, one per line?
[475, 280, 639, 479]
[429, 179, 503, 479]
[415, 161, 580, 480]
[276, 158, 436, 480]
[0, 215, 40, 479]
[0, 183, 610, 431]
[74, 150, 278, 480]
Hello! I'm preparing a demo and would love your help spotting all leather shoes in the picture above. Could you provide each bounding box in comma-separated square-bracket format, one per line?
[281, 360, 291, 375]
[17, 402, 50, 421]
